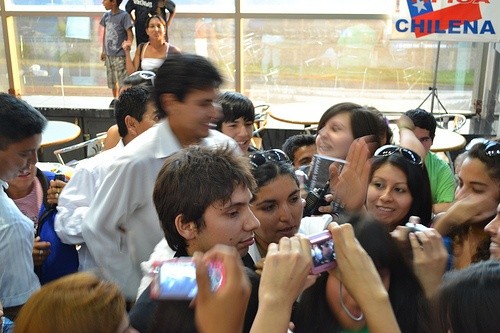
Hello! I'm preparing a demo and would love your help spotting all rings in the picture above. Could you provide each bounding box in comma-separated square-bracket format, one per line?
[38, 255, 42, 261]
[56, 187, 60, 193]
[55, 192, 59, 198]
[40, 249, 43, 255]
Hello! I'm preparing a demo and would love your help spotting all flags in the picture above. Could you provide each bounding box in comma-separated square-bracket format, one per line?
[387, 0, 500, 42]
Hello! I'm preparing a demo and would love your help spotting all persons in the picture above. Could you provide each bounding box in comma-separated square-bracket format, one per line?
[190, 17, 285, 85]
[483, 201, 500, 261]
[122, 143, 261, 333]
[125, 0, 176, 70]
[13, 243, 251, 333]
[80, 53, 243, 315]
[364, 144, 433, 267]
[121, 14, 180, 73]
[282, 134, 317, 169]
[250, 219, 500, 333]
[54, 84, 160, 272]
[403, 107, 456, 215]
[363, 105, 426, 167]
[428, 140, 500, 272]
[241, 147, 303, 333]
[123, 69, 159, 91]
[0, 90, 48, 322]
[98, 0, 134, 107]
[315, 101, 383, 161]
[2, 148, 80, 285]
[291, 218, 436, 333]
[209, 90, 266, 168]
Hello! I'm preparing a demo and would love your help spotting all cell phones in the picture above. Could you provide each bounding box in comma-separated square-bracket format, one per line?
[406, 222, 430, 253]
[54, 174, 65, 189]
[149, 256, 227, 301]
[308, 228, 338, 275]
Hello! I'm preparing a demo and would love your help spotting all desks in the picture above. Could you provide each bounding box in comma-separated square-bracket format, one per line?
[268, 100, 335, 124]
[387, 123, 468, 177]
[36, 121, 82, 150]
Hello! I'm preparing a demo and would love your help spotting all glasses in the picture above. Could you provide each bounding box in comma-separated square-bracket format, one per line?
[373, 144, 423, 165]
[249, 149, 291, 168]
[129, 70, 157, 88]
[465, 137, 500, 159]
